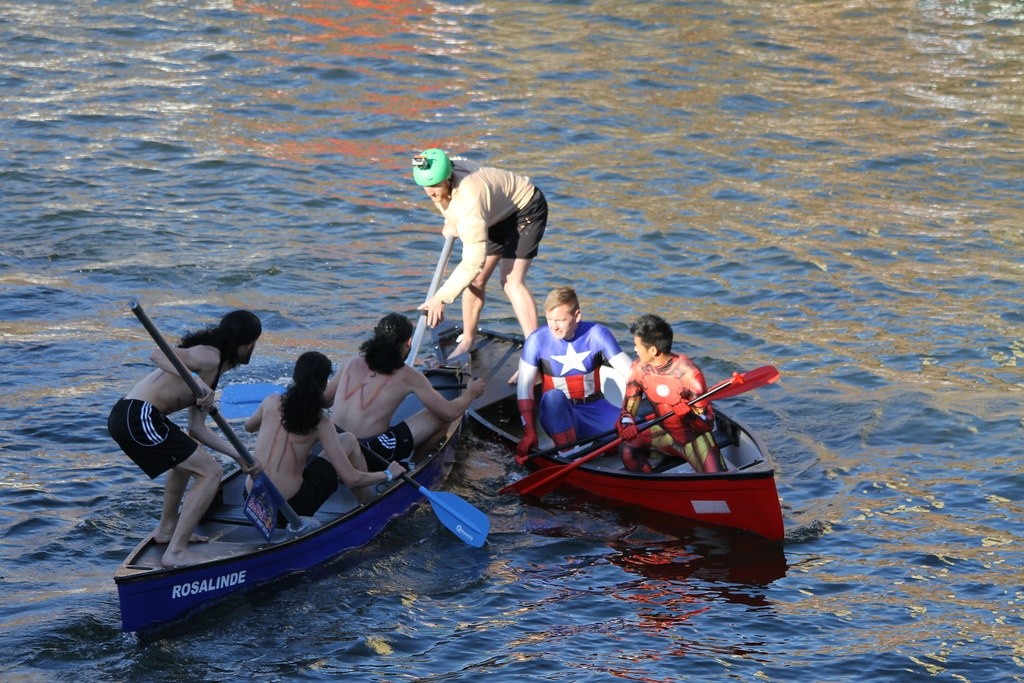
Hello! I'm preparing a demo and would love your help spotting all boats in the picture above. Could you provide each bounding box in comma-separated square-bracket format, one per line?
[113, 351, 472, 634]
[431, 321, 785, 540]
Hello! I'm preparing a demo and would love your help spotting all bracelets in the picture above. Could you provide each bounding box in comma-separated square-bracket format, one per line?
[191, 372, 197, 377]
[384, 470, 392, 481]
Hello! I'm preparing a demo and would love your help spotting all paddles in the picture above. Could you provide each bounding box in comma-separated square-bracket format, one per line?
[515, 363, 779, 465]
[219, 375, 479, 418]
[335, 424, 489, 548]
[497, 372, 743, 496]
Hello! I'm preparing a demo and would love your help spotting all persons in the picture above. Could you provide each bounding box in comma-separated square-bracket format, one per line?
[107, 310, 262, 566]
[616, 314, 728, 474]
[411, 148, 549, 383]
[243, 351, 406, 529]
[515, 287, 633, 457]
[323, 312, 485, 473]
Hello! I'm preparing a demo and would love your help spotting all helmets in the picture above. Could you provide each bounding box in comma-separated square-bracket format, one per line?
[412, 149, 451, 187]
